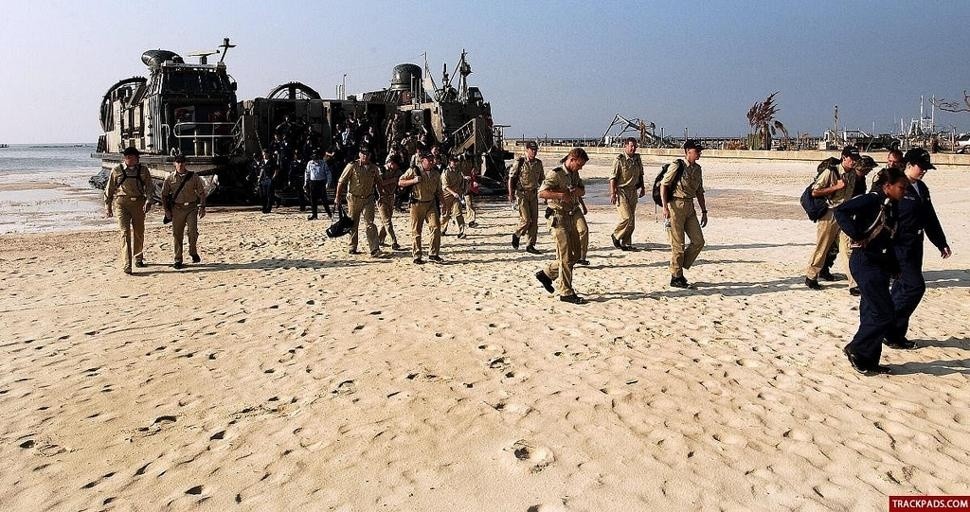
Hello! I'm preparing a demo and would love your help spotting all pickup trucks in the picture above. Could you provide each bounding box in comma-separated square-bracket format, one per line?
[956, 135, 970, 153]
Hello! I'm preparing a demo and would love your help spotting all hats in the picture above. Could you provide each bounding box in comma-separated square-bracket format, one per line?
[842, 145, 862, 163]
[119, 146, 145, 157]
[355, 139, 467, 173]
[683, 139, 704, 150]
[906, 149, 938, 170]
[525, 140, 541, 150]
[173, 154, 187, 163]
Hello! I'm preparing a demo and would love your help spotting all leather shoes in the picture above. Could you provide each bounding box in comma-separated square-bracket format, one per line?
[882, 335, 921, 350]
[849, 286, 861, 297]
[817, 269, 835, 281]
[842, 342, 878, 376]
[804, 277, 822, 291]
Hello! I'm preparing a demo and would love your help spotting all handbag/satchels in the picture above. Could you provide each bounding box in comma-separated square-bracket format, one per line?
[163, 200, 175, 224]
[799, 181, 830, 223]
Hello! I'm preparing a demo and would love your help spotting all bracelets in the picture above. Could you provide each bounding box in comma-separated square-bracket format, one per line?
[702, 210, 707, 213]
[642, 187, 645, 189]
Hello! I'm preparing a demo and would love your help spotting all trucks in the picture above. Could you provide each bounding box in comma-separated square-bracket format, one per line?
[855, 133, 900, 152]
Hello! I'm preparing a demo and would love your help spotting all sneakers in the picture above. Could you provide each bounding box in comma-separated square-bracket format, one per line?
[412, 258, 426, 264]
[380, 241, 391, 248]
[308, 213, 318, 220]
[526, 246, 542, 255]
[669, 274, 690, 289]
[123, 262, 134, 274]
[135, 260, 144, 268]
[173, 261, 184, 270]
[370, 250, 391, 259]
[391, 242, 402, 249]
[511, 232, 521, 251]
[348, 248, 362, 255]
[427, 254, 444, 263]
[610, 233, 621, 249]
[577, 259, 591, 266]
[188, 253, 201, 265]
[622, 245, 637, 251]
[441, 217, 479, 239]
[534, 269, 555, 295]
[560, 294, 586, 304]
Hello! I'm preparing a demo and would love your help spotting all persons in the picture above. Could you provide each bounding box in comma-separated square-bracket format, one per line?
[660, 139, 707, 288]
[609, 138, 645, 250]
[536, 148, 589, 303]
[103, 148, 154, 275]
[507, 142, 547, 254]
[161, 154, 206, 269]
[805, 146, 952, 374]
[254, 115, 478, 263]
[560, 155, 590, 265]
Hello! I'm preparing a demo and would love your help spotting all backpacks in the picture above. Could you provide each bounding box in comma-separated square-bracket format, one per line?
[324, 199, 354, 238]
[394, 165, 422, 202]
[837, 189, 900, 263]
[651, 159, 685, 209]
